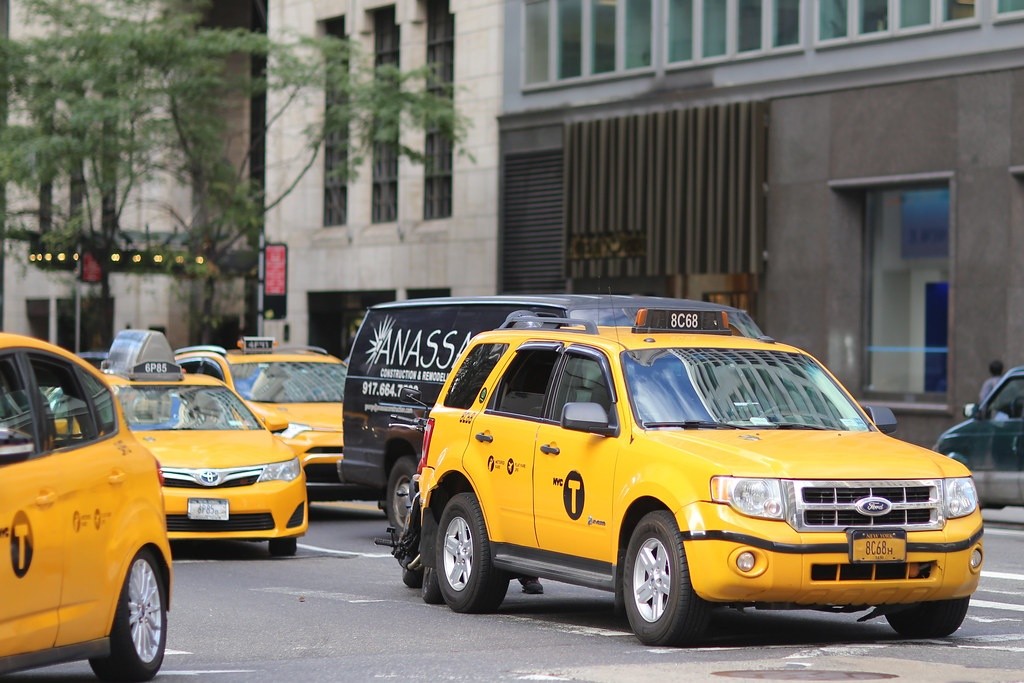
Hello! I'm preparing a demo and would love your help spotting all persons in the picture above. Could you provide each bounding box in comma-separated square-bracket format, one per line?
[978, 359, 1005, 404]
[520, 575, 545, 594]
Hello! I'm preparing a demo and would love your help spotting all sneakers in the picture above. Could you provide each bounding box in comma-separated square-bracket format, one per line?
[522, 576, 544, 593]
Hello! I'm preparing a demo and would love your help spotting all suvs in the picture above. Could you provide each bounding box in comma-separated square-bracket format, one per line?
[335, 294, 766, 542]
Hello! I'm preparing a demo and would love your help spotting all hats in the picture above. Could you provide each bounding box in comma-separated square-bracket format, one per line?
[506, 310, 538, 319]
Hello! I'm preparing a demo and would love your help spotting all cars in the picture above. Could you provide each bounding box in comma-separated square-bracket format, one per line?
[408, 306, 987, 649]
[169, 335, 381, 503]
[0, 327, 174, 683]
[44, 328, 309, 557]
[932, 365, 1024, 513]
